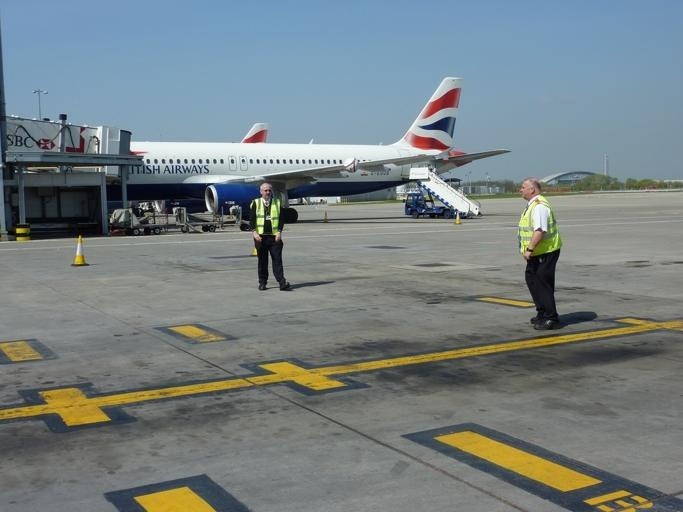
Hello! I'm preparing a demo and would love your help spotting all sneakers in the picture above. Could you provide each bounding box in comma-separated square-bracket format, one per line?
[259, 284, 266, 290]
[280, 282, 289, 290]
[531, 315, 561, 330]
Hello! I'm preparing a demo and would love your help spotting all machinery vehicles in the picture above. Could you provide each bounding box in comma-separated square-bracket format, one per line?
[401, 189, 453, 219]
[107, 207, 222, 235]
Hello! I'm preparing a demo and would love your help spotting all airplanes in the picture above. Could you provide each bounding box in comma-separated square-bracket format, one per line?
[26, 74, 513, 232]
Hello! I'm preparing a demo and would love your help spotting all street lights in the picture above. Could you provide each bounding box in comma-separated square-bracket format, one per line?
[31, 88, 49, 119]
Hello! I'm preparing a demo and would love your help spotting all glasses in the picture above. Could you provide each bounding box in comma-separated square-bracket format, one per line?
[264, 190, 271, 192]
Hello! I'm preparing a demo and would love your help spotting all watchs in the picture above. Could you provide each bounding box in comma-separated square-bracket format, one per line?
[526, 247, 533, 252]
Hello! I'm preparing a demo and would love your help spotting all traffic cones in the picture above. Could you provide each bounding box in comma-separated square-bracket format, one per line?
[251, 248, 258, 255]
[69, 234, 90, 267]
[453, 211, 462, 224]
[322, 207, 328, 223]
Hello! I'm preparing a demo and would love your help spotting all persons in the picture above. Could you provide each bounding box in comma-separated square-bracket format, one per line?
[248, 183, 290, 291]
[516, 177, 563, 330]
